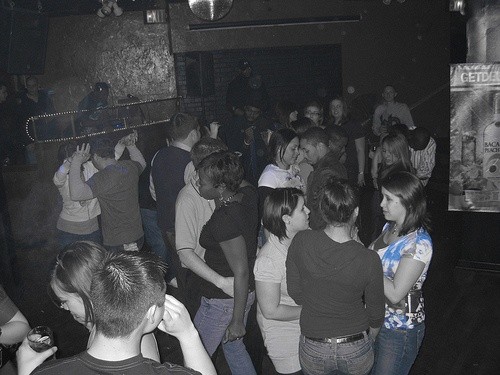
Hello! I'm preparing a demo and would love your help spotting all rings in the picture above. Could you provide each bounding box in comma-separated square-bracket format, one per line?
[237, 338, 240, 341]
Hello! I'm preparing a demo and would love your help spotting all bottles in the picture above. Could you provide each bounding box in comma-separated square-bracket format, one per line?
[483, 93, 500, 205]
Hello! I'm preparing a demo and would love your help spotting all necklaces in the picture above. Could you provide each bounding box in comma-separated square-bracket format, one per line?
[392, 223, 401, 236]
[215, 192, 241, 207]
[189, 179, 214, 214]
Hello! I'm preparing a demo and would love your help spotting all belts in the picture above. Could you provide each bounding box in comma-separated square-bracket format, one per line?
[305, 328, 370, 344]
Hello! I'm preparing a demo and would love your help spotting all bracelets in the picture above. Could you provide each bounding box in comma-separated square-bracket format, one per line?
[67, 158, 71, 164]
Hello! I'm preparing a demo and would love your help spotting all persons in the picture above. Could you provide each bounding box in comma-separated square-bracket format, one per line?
[16, 252, 218, 375]
[19, 75, 62, 142]
[252, 187, 313, 375]
[47, 240, 161, 363]
[174, 137, 261, 374]
[287, 174, 384, 374]
[226, 60, 273, 116]
[148, 113, 202, 306]
[68, 138, 144, 253]
[52, 134, 136, 246]
[141, 84, 440, 262]
[78, 83, 116, 146]
[1, 83, 27, 165]
[0, 144, 65, 289]
[0, 279, 32, 375]
[195, 150, 257, 375]
[366, 171, 434, 375]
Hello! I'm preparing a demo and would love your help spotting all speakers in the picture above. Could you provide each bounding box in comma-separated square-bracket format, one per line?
[0, 6, 49, 76]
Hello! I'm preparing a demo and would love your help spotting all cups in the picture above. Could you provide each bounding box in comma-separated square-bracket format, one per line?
[27, 326, 56, 364]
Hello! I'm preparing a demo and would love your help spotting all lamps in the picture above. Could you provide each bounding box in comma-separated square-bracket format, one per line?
[96, 0, 123, 18]
